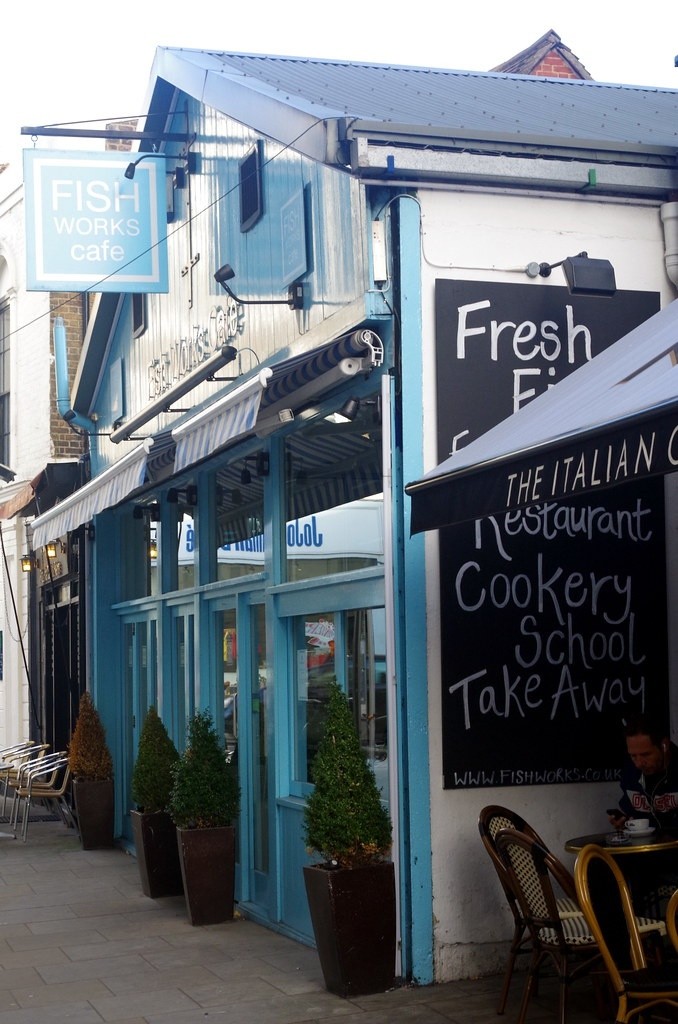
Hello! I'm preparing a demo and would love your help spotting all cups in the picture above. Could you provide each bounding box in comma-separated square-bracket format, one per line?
[625, 819, 649, 830]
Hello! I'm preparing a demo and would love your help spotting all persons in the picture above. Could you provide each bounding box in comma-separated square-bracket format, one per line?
[587, 714, 678, 1004]
[224, 682, 230, 696]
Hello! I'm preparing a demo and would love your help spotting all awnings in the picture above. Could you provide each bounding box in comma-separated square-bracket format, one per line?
[31, 429, 177, 552]
[171, 330, 373, 473]
[177, 398, 382, 551]
[405, 297, 678, 539]
[0, 462, 85, 520]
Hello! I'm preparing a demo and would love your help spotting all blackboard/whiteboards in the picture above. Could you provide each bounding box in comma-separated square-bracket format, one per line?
[436, 281, 670, 788]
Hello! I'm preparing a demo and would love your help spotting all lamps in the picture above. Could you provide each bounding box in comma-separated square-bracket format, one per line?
[214, 264, 303, 310]
[257, 452, 270, 476]
[333, 395, 381, 424]
[19, 554, 40, 572]
[125, 152, 201, 180]
[109, 346, 237, 442]
[187, 485, 198, 505]
[538, 251, 617, 298]
[149, 503, 159, 522]
[133, 505, 144, 520]
[46, 538, 66, 559]
[278, 401, 321, 422]
[167, 488, 186, 503]
[241, 456, 257, 485]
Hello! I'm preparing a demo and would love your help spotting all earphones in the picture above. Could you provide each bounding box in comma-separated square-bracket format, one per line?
[663, 744, 666, 752]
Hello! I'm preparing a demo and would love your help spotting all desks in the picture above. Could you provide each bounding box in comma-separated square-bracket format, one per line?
[0, 762, 14, 770]
[565, 830, 678, 854]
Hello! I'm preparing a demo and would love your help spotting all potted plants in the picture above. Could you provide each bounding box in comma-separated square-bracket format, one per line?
[166, 706, 242, 926]
[129, 706, 186, 899]
[69, 692, 114, 850]
[302, 675, 396, 998]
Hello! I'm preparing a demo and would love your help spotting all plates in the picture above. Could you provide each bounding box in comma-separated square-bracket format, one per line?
[623, 828, 655, 837]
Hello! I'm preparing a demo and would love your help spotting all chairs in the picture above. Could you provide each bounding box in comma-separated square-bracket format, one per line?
[574, 843, 678, 1024]
[479, 804, 575, 1016]
[0, 741, 78, 844]
[495, 828, 666, 1024]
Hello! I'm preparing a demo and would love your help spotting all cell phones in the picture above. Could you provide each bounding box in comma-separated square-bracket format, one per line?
[606, 809, 630, 822]
